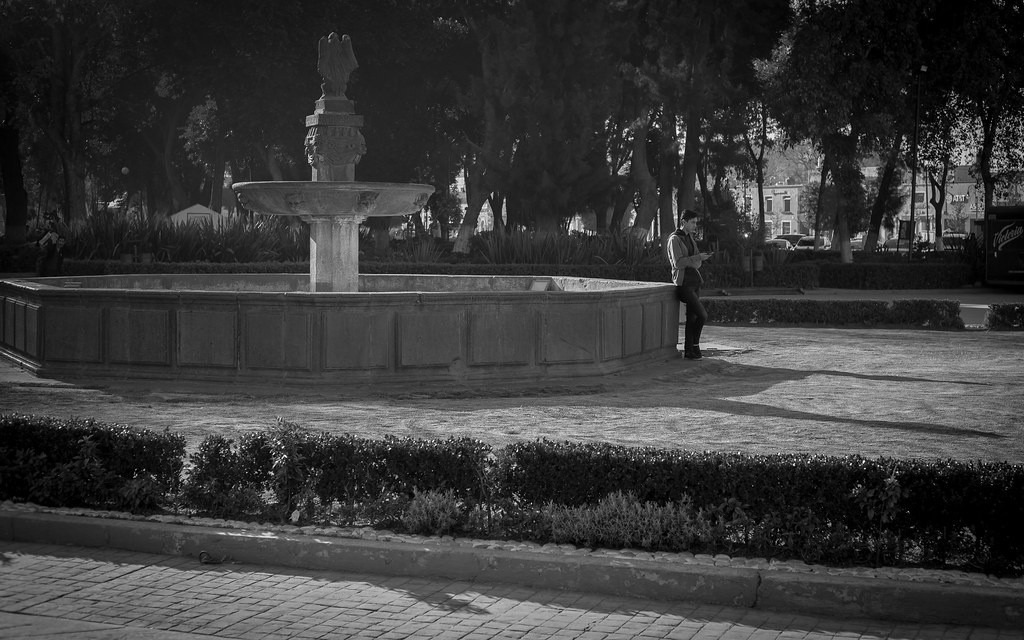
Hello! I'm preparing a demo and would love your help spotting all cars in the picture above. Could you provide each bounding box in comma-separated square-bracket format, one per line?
[776, 233, 808, 246]
[884, 234, 911, 248]
[849, 238, 864, 251]
[764, 240, 794, 251]
[794, 236, 833, 254]
[942, 231, 968, 252]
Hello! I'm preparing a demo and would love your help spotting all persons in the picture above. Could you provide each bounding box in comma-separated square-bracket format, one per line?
[667, 209, 712, 360]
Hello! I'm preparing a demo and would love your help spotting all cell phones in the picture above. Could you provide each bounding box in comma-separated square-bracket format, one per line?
[708, 252, 714, 255]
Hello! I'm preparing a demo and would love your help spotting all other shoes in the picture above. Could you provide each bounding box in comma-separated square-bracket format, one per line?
[684, 344, 703, 361]
